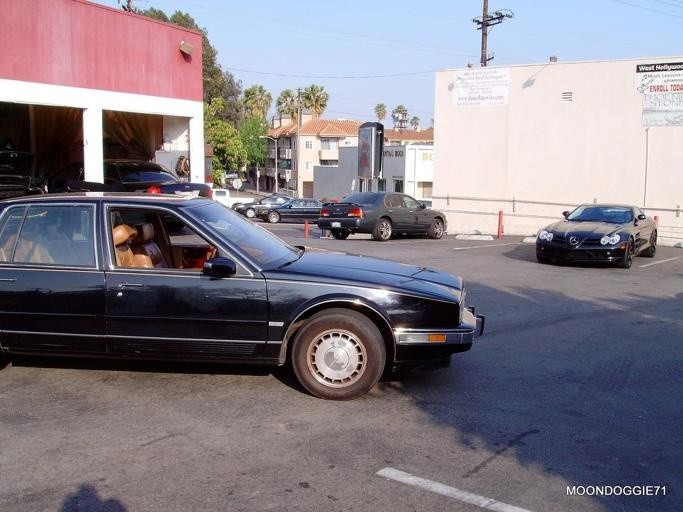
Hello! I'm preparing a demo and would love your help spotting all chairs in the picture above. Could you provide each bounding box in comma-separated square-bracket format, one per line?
[113, 220, 169, 269]
[0, 221, 78, 266]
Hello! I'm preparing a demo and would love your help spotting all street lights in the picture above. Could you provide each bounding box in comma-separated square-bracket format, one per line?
[259, 135, 278, 194]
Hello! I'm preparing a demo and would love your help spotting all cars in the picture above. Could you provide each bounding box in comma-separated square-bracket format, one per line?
[66, 160, 212, 197]
[0, 149, 50, 200]
[535, 203, 658, 269]
